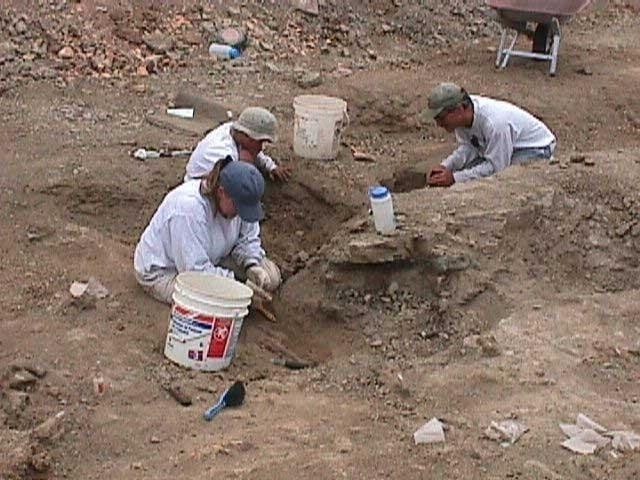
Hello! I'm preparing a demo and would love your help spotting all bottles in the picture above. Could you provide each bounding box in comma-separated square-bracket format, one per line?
[370, 186, 398, 232]
[209, 42, 238, 61]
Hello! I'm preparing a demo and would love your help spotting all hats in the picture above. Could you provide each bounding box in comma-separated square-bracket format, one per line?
[231, 106, 278, 143]
[419, 83, 465, 124]
[220, 161, 265, 222]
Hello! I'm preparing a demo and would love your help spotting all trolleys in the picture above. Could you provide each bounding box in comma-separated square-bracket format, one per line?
[484, 0, 591, 78]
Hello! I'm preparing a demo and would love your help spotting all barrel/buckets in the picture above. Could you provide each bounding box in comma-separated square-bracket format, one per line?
[292, 94, 349, 159]
[164, 270, 253, 371]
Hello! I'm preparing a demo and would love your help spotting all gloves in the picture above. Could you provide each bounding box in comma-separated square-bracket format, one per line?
[245, 265, 276, 323]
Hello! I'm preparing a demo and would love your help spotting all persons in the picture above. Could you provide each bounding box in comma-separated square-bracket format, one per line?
[133, 157, 274, 312]
[185, 105, 291, 191]
[425, 84, 557, 188]
